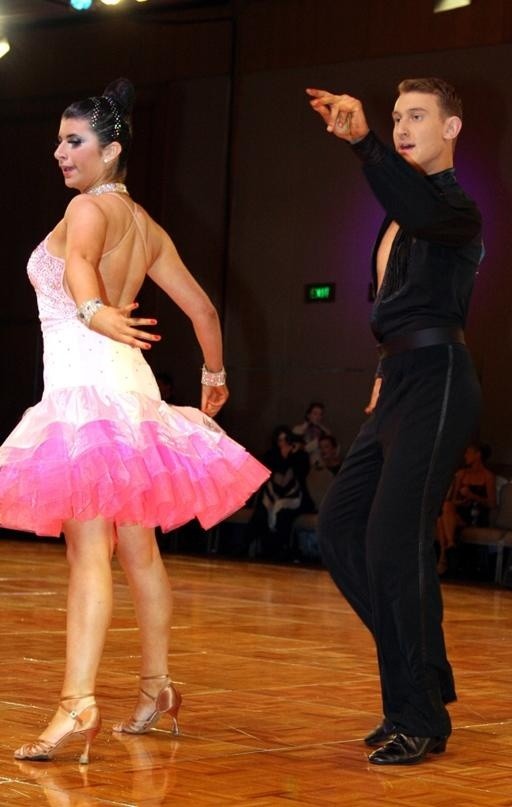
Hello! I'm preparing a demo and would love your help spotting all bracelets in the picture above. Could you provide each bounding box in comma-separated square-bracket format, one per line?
[74, 297, 105, 328]
[201, 363, 228, 387]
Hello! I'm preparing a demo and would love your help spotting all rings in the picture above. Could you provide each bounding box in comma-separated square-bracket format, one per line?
[208, 404, 214, 409]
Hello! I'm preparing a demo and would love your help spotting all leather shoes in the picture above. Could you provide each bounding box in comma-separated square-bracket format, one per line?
[366, 717, 446, 764]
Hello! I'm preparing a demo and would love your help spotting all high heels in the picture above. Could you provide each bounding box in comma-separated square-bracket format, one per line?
[113, 676, 182, 736]
[14, 694, 101, 764]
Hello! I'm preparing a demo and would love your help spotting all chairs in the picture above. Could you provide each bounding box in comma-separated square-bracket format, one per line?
[449, 520, 511, 586]
[180, 502, 327, 571]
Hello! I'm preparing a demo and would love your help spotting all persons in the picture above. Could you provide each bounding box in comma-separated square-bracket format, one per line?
[1, 78, 231, 761]
[308, 435, 346, 511]
[290, 405, 333, 456]
[434, 439, 497, 576]
[260, 428, 311, 541]
[303, 75, 481, 765]
[14, 732, 182, 805]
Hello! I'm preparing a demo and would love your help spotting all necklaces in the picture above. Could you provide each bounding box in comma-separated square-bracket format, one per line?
[86, 182, 130, 198]
